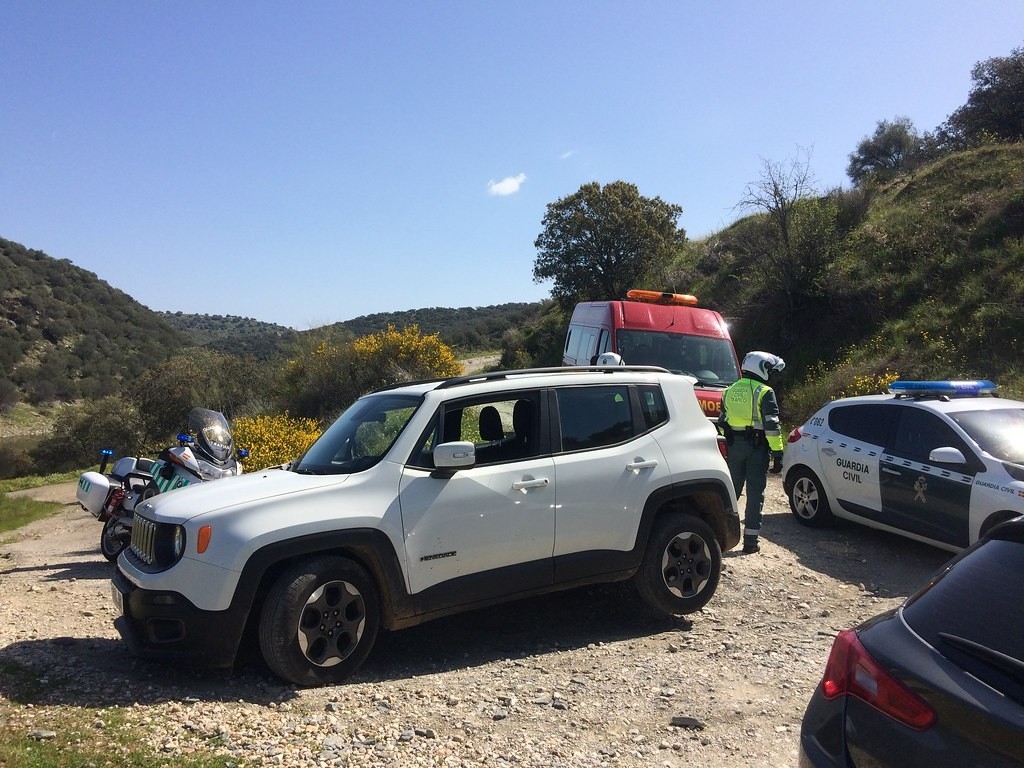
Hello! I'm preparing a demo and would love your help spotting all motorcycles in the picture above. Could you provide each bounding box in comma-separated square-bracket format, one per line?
[77, 407, 248, 562]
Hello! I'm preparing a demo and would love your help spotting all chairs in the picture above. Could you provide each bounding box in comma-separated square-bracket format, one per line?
[478, 407, 504, 462]
[627, 343, 651, 364]
[669, 341, 700, 368]
[513, 400, 534, 458]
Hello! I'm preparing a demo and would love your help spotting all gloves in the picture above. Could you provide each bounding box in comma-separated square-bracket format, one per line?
[769, 450, 783, 474]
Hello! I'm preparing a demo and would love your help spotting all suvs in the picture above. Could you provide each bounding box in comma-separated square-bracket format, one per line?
[111, 365, 740, 686]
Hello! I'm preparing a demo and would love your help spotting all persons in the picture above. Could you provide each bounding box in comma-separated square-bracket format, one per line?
[718, 352, 785, 553]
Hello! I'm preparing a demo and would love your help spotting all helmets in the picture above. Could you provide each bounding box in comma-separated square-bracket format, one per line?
[741, 351, 785, 382]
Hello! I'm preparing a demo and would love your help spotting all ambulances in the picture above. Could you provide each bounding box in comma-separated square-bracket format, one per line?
[562, 290, 742, 462]
[781, 380, 1024, 551]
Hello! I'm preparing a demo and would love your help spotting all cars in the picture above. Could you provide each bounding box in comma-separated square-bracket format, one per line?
[799, 515, 1024, 768]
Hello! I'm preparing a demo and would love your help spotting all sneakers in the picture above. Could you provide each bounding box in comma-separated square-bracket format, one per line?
[743, 539, 760, 554]
